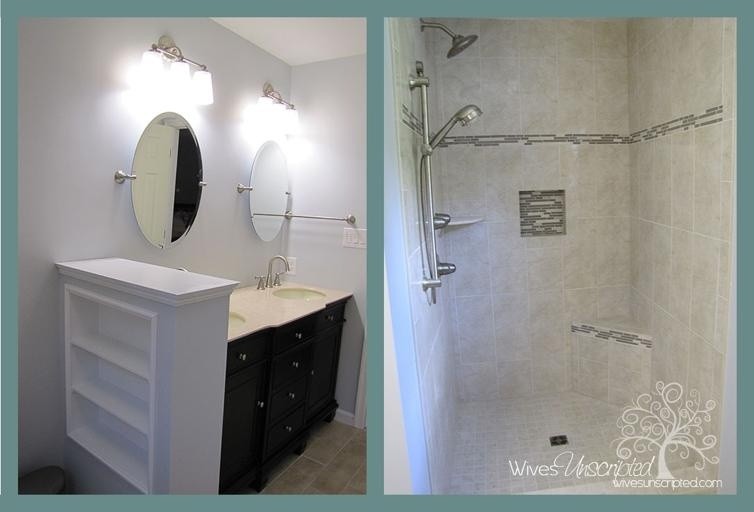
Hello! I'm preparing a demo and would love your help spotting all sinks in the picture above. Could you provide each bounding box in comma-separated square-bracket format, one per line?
[272, 288, 326, 302]
[228, 309, 246, 332]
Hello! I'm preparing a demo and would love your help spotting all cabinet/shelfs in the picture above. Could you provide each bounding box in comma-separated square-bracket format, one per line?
[58, 275, 230, 494]
[218, 303, 345, 494]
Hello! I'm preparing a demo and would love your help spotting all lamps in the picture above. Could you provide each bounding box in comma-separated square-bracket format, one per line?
[259, 82, 299, 122]
[143, 35, 214, 106]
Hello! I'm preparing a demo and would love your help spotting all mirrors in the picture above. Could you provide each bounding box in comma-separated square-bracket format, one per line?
[130, 111, 203, 250]
[248, 140, 288, 241]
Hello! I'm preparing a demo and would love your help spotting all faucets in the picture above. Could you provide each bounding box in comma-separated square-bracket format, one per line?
[178, 268, 190, 274]
[267, 256, 290, 288]
